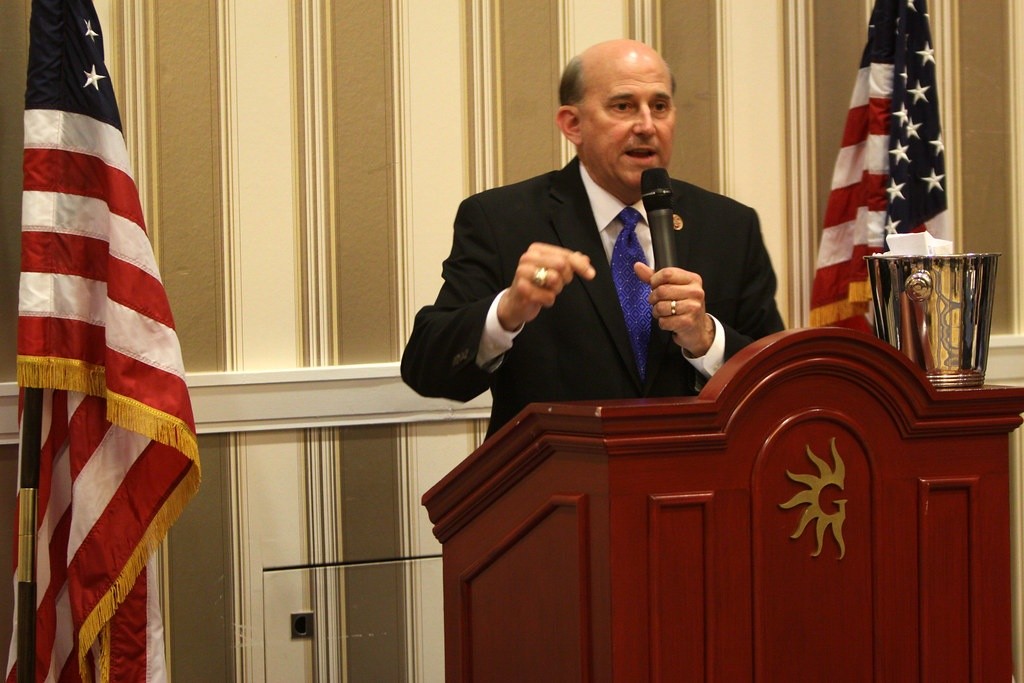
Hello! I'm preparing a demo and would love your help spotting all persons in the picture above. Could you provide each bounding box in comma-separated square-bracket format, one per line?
[400, 38, 787, 441]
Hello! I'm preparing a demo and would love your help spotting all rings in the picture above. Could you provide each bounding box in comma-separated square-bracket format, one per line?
[670, 300, 677, 315]
[530, 266, 549, 289]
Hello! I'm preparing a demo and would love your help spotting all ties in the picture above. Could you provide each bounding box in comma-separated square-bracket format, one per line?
[611, 207, 653, 385]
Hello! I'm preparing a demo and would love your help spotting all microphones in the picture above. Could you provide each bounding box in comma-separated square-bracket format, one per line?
[641, 168, 677, 271]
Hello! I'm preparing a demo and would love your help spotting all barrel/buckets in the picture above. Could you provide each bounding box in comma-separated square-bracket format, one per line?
[865, 254, 1002, 387]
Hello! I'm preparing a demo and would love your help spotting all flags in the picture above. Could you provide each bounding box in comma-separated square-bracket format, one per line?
[3, 0, 202, 683]
[806, 0, 956, 337]
